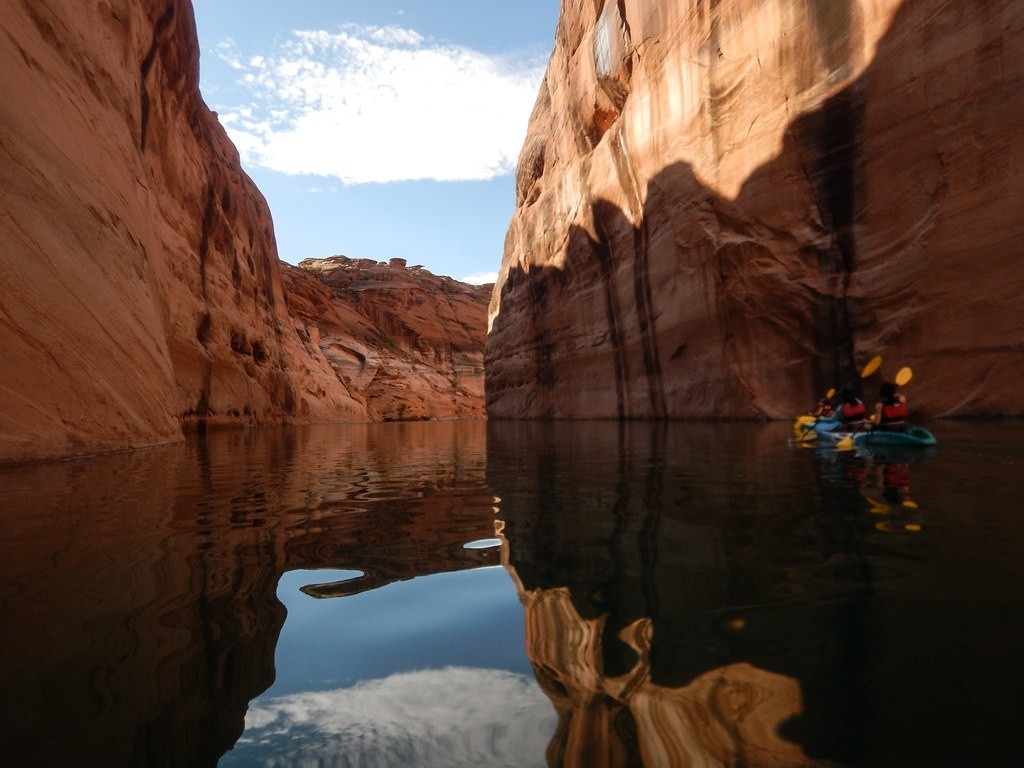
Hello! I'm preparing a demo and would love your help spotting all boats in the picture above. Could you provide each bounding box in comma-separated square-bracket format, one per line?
[796, 414, 938, 447]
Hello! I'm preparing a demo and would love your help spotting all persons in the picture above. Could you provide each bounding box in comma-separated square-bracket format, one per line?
[878, 374, 896, 407]
[808, 389, 859, 440]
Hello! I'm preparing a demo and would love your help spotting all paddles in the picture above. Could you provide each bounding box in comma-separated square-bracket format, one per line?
[795, 354, 882, 441]
[836, 367, 912, 449]
